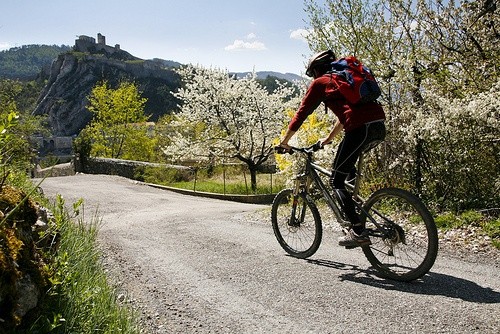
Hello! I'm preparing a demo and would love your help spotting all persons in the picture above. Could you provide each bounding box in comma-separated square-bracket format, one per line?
[276, 50, 386, 248]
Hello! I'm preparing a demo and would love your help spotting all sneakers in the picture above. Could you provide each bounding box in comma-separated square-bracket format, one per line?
[338, 228, 372, 246]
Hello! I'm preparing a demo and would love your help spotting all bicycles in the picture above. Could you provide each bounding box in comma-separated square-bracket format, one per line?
[270, 140, 438, 282]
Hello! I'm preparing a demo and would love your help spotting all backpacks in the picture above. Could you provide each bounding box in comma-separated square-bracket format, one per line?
[329, 56, 381, 107]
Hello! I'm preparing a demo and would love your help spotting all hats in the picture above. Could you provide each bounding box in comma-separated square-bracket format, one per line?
[306, 50, 336, 77]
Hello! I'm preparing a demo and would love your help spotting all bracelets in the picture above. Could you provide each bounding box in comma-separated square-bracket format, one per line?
[280, 141, 287, 145]
[326, 137, 330, 143]
